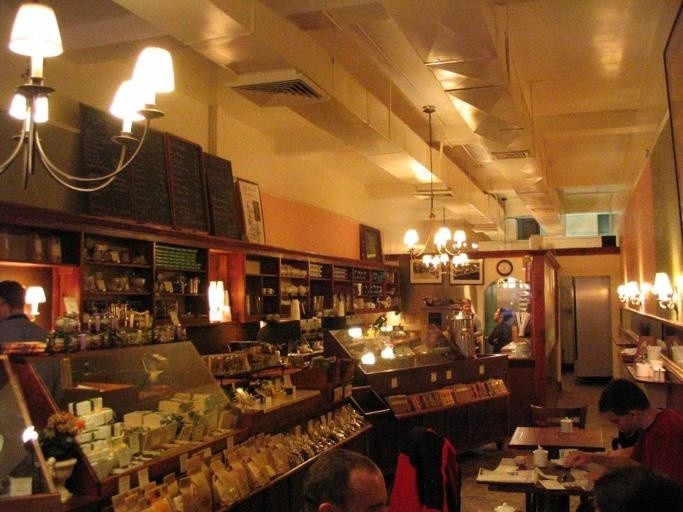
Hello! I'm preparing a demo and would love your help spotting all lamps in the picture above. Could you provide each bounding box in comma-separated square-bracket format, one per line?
[614, 279, 642, 311]
[649, 269, 683, 321]
[0, 1, 177, 195]
[403, 101, 469, 260]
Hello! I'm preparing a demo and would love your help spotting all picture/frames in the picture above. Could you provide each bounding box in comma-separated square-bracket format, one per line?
[449, 258, 484, 285]
[360, 223, 382, 264]
[409, 258, 444, 285]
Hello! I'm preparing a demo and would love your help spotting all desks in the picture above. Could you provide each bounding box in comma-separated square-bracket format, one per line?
[487, 452, 609, 511]
[508, 425, 609, 456]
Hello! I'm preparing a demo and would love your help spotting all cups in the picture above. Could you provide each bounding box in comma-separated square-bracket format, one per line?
[560, 420, 574, 432]
[633, 344, 667, 377]
[532, 450, 549, 468]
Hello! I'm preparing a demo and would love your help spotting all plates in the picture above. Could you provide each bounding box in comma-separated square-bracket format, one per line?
[551, 458, 563, 467]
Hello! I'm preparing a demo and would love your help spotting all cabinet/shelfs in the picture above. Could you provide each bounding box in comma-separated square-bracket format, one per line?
[322, 306, 510, 456]
[281, 308, 396, 509]
[504, 253, 561, 428]
[280, 249, 404, 332]
[1, 201, 280, 508]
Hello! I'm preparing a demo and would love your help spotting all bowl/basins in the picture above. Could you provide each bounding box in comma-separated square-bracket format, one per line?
[558, 449, 579, 463]
[133, 278, 146, 286]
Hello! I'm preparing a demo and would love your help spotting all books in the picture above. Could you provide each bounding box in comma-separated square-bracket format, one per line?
[476, 467, 538, 486]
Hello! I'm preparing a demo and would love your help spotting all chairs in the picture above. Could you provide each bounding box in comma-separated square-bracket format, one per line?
[522, 402, 590, 511]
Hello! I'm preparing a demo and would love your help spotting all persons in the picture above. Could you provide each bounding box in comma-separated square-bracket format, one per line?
[589, 465, 683, 512]
[0, 280, 49, 344]
[303, 449, 387, 512]
[484, 307, 512, 354]
[563, 377, 683, 481]
[455, 298, 483, 358]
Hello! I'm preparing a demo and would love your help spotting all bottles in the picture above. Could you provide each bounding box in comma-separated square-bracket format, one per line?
[319, 301, 345, 318]
[46, 312, 187, 355]
[514, 454, 526, 466]
[289, 299, 301, 319]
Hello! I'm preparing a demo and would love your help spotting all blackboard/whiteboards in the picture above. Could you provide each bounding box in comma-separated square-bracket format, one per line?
[80, 103, 241, 241]
